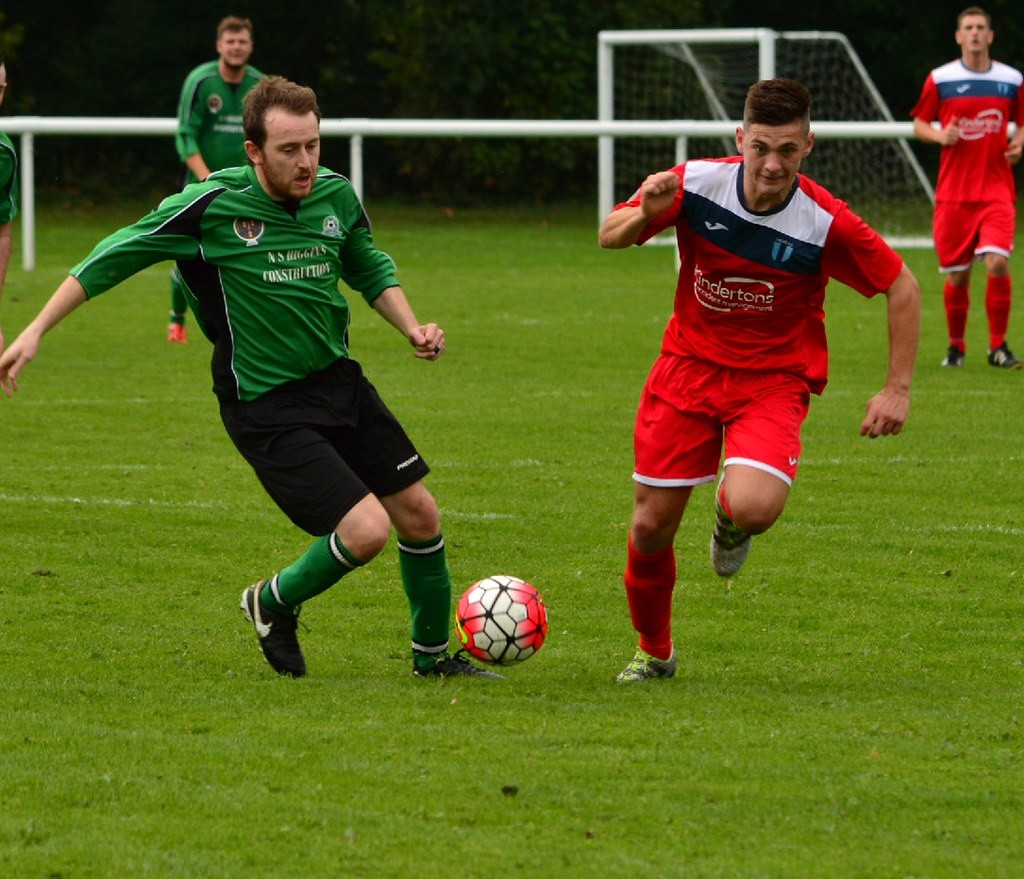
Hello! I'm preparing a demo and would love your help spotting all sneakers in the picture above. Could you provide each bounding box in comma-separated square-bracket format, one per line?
[709, 472, 752, 577]
[940, 345, 966, 369]
[239, 579, 307, 680]
[615, 638, 678, 685]
[986, 341, 1024, 371]
[167, 322, 190, 345]
[410, 654, 507, 680]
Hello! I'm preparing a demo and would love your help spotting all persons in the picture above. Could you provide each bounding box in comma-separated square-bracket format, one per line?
[0, 59, 18, 357]
[0, 77, 504, 677]
[598, 78, 923, 679]
[166, 17, 266, 341]
[909, 7, 1023, 369]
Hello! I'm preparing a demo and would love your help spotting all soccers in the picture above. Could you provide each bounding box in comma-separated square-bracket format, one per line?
[458, 575, 546, 667]
